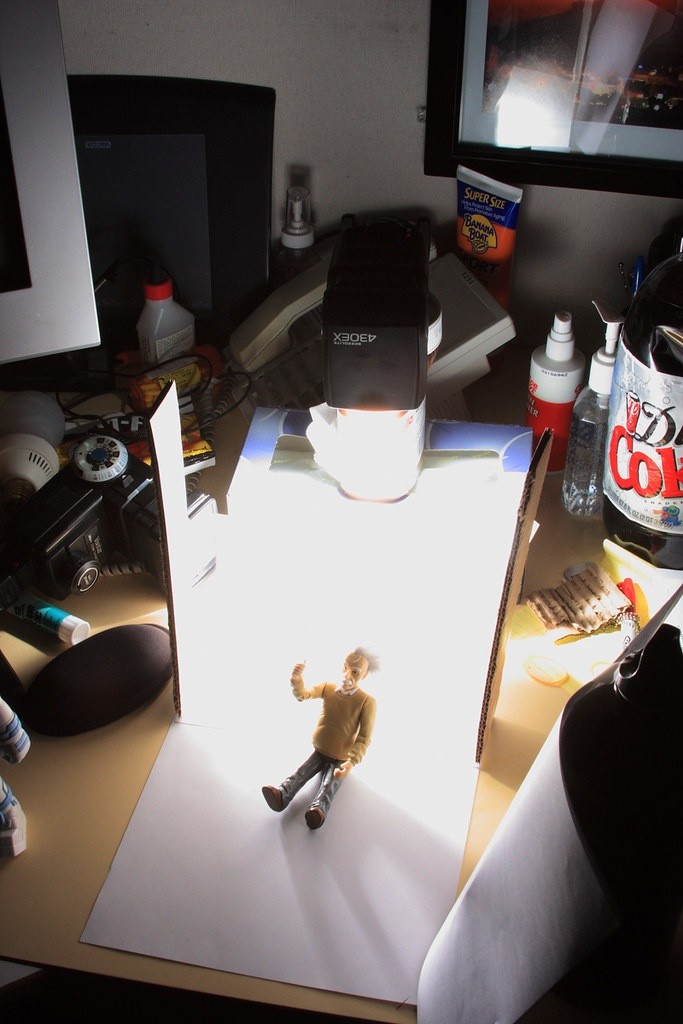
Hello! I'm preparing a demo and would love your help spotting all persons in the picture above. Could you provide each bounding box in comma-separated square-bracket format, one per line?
[263, 646, 376, 831]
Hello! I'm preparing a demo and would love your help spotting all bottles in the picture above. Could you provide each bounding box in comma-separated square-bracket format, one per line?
[277, 185, 315, 276]
[602, 236, 683, 573]
[138, 276, 196, 364]
[525, 308, 586, 475]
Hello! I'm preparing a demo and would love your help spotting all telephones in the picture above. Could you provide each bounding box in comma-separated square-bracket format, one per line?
[223, 228, 516, 427]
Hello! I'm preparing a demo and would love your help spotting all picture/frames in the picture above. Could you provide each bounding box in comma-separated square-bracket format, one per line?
[424, 0, 683, 199]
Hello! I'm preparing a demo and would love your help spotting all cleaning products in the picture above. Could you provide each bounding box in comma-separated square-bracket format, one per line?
[559, 298, 629, 518]
[526, 311, 589, 476]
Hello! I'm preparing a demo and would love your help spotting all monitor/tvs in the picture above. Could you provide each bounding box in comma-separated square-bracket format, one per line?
[0, 0, 101, 449]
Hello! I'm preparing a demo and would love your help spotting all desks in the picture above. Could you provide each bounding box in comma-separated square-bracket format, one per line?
[0, 343, 683, 1024]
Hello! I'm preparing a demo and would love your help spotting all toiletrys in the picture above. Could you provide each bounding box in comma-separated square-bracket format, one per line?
[0, 576, 91, 647]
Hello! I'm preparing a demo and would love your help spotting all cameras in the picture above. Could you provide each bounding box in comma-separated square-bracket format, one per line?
[0, 434, 221, 613]
